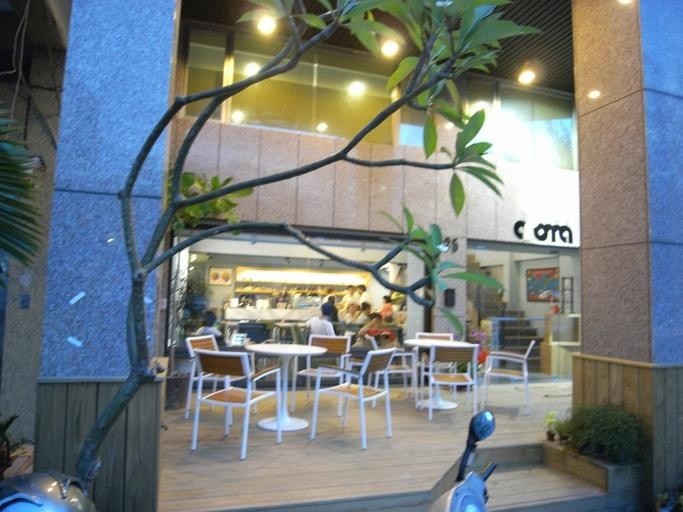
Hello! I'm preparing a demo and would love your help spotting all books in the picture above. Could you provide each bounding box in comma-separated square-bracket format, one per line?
[232, 332, 248, 345]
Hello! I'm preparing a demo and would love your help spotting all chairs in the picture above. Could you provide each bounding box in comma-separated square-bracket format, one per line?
[190, 347, 283, 460]
[479, 338, 534, 416]
[420, 342, 479, 420]
[364, 333, 418, 409]
[185, 335, 258, 425]
[308, 347, 396, 450]
[291, 333, 350, 415]
[417, 331, 457, 401]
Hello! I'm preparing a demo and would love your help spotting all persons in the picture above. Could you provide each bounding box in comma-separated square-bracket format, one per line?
[322, 295, 338, 321]
[359, 313, 382, 333]
[196, 311, 218, 336]
[340, 284, 372, 322]
[378, 295, 393, 320]
[304, 302, 337, 337]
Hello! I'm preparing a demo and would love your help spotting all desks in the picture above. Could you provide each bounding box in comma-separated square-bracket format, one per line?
[245, 343, 325, 431]
[404, 338, 470, 410]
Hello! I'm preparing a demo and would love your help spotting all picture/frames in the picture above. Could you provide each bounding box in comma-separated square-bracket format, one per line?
[208, 266, 233, 286]
[526, 268, 560, 302]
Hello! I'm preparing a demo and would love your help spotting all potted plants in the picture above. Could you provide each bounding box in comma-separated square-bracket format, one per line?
[542, 411, 558, 441]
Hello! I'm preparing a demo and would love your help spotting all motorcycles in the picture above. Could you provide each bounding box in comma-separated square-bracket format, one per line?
[1, 406, 500, 511]
